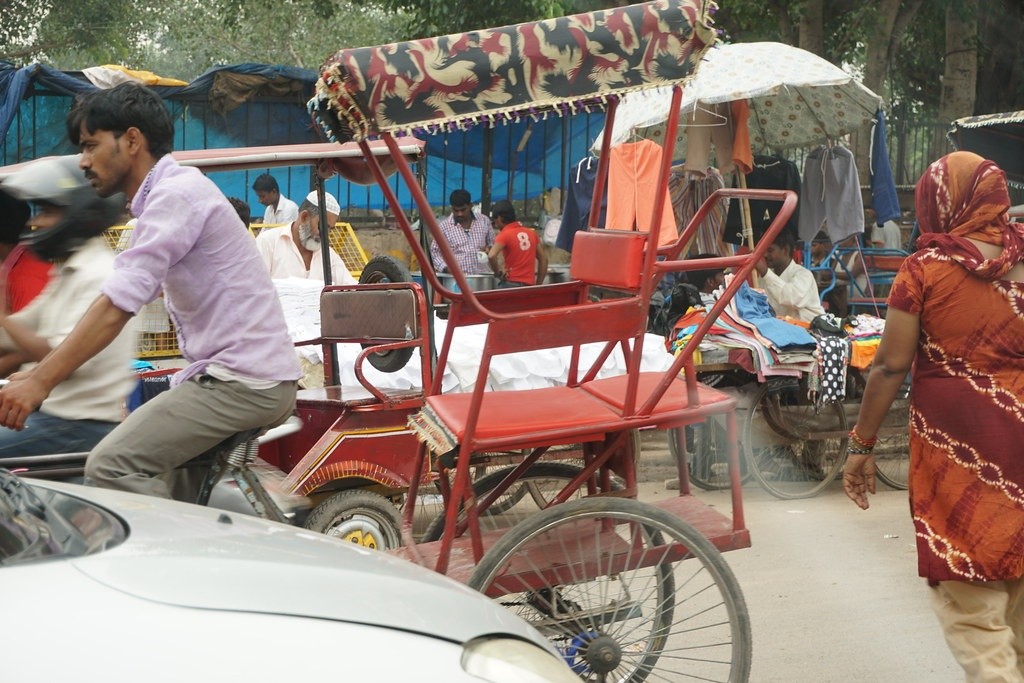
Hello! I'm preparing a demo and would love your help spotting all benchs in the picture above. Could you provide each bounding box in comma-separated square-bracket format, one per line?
[845, 220, 922, 305]
[407, 223, 644, 510]
[288, 284, 429, 401]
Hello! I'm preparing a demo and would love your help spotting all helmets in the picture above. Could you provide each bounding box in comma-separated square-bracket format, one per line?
[0, 154, 127, 261]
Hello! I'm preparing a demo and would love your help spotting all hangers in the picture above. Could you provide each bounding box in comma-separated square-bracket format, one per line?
[752, 136, 851, 164]
[614, 129, 658, 148]
[677, 101, 727, 127]
[581, 139, 603, 159]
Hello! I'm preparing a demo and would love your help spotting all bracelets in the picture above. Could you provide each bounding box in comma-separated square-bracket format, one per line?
[847, 424, 878, 453]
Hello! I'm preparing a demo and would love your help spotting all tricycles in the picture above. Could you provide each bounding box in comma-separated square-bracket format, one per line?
[1, 0, 762, 683]
[666, 332, 914, 502]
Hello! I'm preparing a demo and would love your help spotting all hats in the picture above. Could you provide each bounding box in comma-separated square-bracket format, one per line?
[306, 190, 340, 216]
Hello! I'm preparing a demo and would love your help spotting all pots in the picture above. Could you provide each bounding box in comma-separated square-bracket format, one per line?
[410, 263, 570, 303]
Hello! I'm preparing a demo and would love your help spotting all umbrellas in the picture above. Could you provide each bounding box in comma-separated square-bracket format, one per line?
[588, 40, 889, 238]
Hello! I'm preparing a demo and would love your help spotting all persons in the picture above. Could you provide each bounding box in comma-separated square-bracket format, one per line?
[803, 208, 901, 318]
[687, 253, 726, 308]
[0, 75, 304, 501]
[0, 152, 135, 471]
[843, 151, 1024, 683]
[481, 199, 554, 295]
[117, 171, 360, 351]
[429, 189, 503, 276]
[751, 226, 827, 324]
